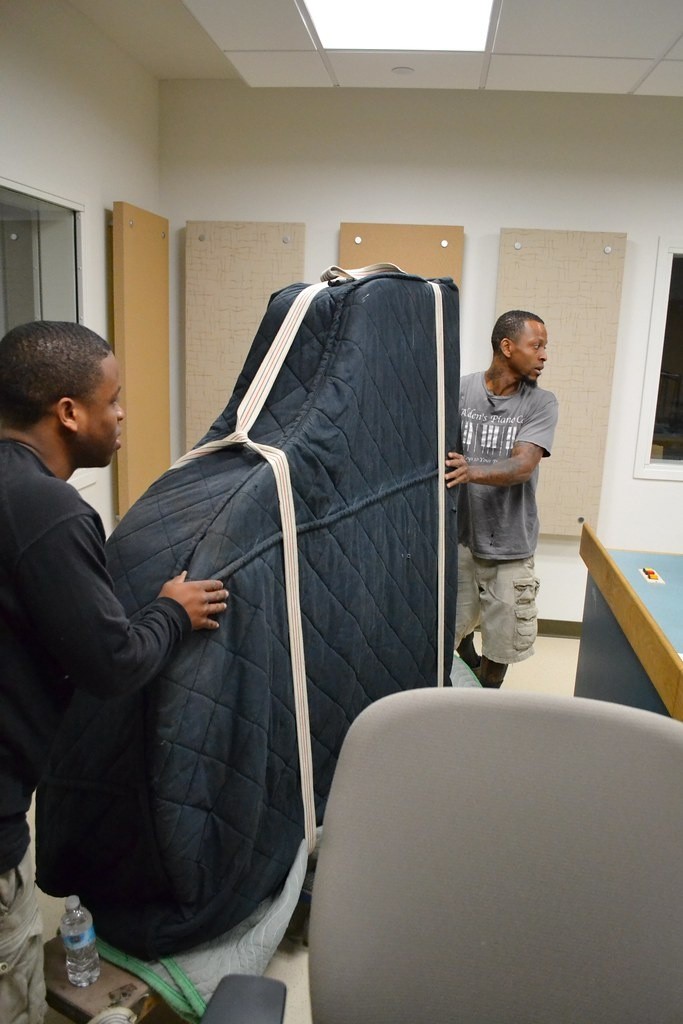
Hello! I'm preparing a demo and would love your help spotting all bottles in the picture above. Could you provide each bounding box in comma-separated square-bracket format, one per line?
[60, 894, 100, 988]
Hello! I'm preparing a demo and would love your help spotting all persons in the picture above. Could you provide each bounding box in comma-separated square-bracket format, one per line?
[1, 320, 229, 1022]
[440, 308, 562, 686]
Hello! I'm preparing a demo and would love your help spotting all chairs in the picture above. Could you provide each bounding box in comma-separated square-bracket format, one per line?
[193, 684, 681, 1024]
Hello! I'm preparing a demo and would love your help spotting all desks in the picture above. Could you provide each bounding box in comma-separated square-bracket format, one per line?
[574, 517, 683, 724]
